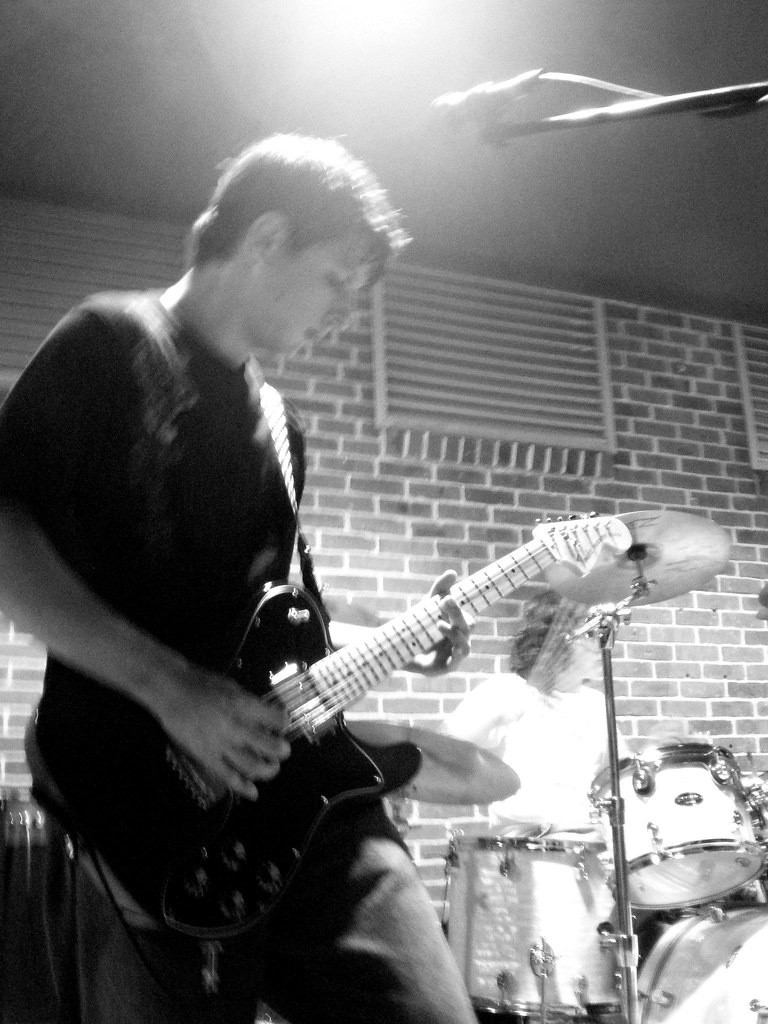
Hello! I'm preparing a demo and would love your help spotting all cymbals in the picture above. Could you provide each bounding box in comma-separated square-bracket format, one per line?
[344, 716, 522, 807]
[543, 507, 734, 608]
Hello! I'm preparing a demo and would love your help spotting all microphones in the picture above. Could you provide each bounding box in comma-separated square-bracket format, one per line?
[428, 69, 549, 130]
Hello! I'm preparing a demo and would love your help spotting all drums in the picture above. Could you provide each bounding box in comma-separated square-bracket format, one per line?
[588, 739, 764, 910]
[606, 913, 768, 1022]
[445, 827, 631, 1014]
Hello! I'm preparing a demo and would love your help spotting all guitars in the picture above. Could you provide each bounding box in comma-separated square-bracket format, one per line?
[21, 509, 634, 939]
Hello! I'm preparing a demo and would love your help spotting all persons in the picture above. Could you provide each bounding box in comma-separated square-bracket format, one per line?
[431, 587, 637, 842]
[1, 127, 472, 1024]
